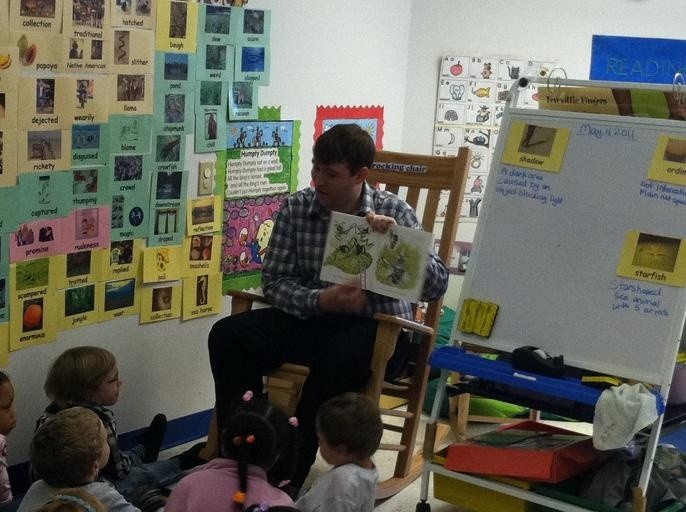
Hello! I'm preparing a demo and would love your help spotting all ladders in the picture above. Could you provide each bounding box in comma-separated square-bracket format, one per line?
[359, 264, 365, 290]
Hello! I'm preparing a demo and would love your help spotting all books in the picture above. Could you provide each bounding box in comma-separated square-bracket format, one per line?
[319, 211, 435, 304]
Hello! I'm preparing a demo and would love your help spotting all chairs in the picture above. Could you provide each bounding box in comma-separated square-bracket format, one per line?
[195, 142, 472, 501]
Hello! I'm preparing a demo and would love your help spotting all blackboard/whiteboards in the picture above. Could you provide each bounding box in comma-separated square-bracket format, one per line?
[450, 107, 686, 386]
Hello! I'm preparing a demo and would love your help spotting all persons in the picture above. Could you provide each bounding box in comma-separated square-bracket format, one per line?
[163, 390, 298, 512]
[208, 124, 450, 480]
[208, 114, 216, 139]
[70, 43, 78, 59]
[295, 392, 383, 512]
[17, 346, 207, 512]
[0, 371, 16, 507]
[37, 83, 46, 111]
[73, 0, 105, 29]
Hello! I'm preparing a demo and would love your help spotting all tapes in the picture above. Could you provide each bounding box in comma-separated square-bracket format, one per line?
[533, 349, 546, 361]
